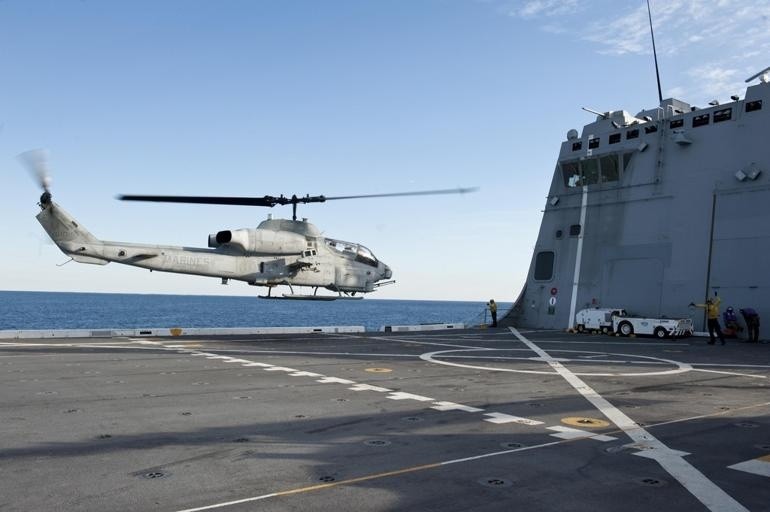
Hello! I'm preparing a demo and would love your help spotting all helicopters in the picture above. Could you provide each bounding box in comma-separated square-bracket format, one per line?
[17, 147, 481, 301]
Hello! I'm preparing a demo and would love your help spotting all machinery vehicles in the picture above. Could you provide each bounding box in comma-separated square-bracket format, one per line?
[575, 307, 627, 335]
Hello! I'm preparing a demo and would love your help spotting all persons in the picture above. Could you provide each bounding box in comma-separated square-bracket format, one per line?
[725, 305, 738, 330]
[486, 298, 498, 328]
[686, 291, 726, 346]
[739, 307, 761, 344]
